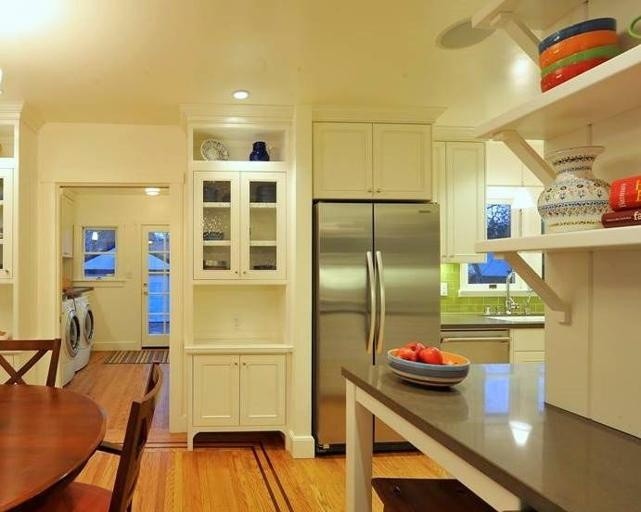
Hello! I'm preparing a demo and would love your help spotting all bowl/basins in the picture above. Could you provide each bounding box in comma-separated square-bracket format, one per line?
[203, 260, 226, 270]
[203, 231, 223, 240]
[384, 348, 471, 390]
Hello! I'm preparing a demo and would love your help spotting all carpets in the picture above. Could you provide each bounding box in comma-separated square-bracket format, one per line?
[104, 351, 169, 364]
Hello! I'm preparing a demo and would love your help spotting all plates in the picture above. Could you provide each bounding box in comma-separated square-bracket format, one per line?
[253, 264, 275, 270]
[203, 183, 229, 202]
[255, 185, 275, 203]
[537, 16, 619, 93]
[200, 138, 229, 161]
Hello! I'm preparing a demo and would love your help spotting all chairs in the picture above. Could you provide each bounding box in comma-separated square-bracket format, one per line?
[34, 360, 163, 512]
[0, 339, 61, 387]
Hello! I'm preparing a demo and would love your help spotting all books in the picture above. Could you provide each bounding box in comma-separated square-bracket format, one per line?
[602, 175, 641, 227]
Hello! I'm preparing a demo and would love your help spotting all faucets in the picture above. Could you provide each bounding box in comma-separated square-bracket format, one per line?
[505, 271, 519, 315]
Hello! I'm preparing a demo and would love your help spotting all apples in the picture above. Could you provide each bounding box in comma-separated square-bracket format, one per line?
[397, 342, 443, 367]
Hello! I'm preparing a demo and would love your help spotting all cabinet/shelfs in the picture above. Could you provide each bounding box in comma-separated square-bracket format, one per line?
[184, 105, 294, 451]
[0, 100, 45, 384]
[312, 106, 447, 200]
[434, 127, 487, 263]
[472, 0, 641, 325]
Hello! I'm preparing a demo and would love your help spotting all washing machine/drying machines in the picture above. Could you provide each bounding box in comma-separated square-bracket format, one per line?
[62, 294, 95, 387]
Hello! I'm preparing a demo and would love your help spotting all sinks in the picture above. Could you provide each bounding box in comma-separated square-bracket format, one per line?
[486, 316, 546, 322]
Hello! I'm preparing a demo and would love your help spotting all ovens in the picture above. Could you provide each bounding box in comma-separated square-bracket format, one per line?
[440, 329, 511, 365]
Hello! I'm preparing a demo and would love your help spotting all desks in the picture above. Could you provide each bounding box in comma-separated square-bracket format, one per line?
[342, 363, 641, 512]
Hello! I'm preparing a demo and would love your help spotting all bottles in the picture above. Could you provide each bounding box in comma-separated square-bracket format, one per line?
[249, 141, 269, 161]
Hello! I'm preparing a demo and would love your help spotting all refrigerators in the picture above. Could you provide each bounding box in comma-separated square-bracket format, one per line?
[313, 200, 443, 456]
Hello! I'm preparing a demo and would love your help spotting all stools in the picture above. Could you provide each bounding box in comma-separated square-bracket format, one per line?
[371, 478, 498, 512]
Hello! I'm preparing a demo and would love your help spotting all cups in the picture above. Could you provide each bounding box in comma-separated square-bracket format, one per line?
[627, 14, 641, 42]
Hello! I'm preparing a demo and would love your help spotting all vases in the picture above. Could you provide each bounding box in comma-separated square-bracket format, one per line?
[537, 146, 611, 233]
